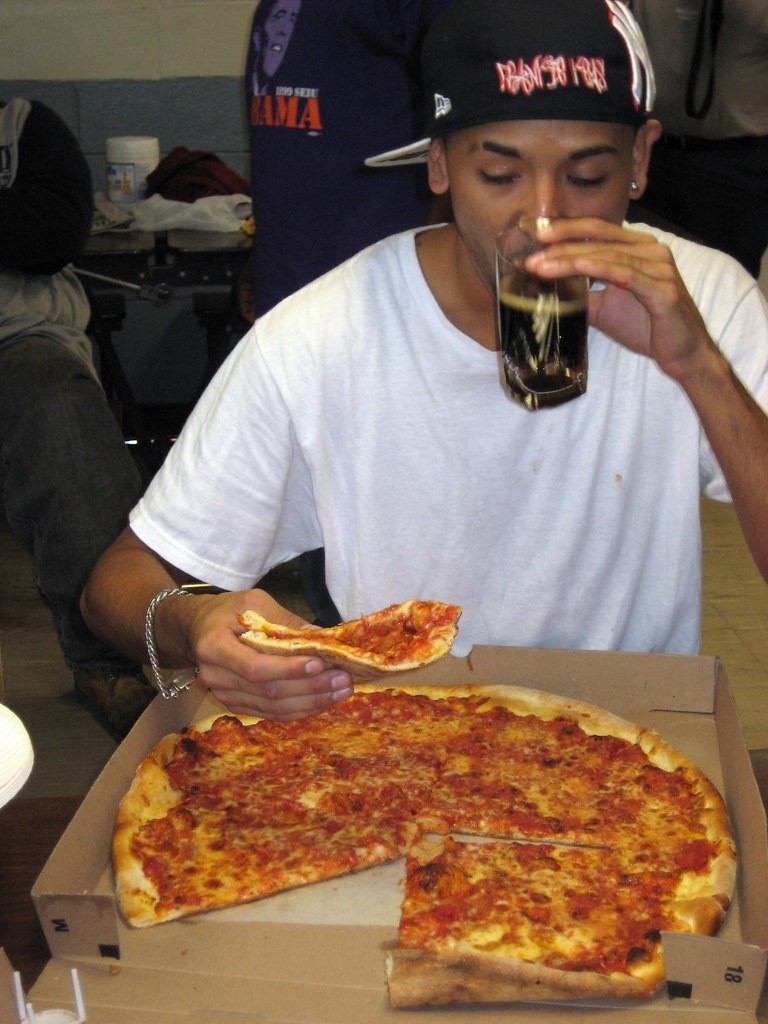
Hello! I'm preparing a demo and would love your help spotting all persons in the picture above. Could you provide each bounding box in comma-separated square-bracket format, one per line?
[83, 0, 768, 722]
[228, 0, 768, 329]
[0, 97, 183, 744]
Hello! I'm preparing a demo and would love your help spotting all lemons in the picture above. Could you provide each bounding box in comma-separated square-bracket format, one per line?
[499, 272, 592, 314]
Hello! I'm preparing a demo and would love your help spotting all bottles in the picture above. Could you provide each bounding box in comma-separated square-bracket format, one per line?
[105, 135, 160, 205]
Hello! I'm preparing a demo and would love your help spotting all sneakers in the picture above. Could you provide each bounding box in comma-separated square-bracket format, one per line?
[71, 669, 161, 739]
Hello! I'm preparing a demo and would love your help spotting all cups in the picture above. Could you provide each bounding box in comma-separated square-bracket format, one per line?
[495, 218, 590, 411]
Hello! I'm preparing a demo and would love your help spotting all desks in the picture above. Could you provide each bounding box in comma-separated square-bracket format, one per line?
[79, 232, 253, 468]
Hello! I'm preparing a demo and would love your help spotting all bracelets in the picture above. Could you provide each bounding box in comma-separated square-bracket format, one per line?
[142, 585, 199, 699]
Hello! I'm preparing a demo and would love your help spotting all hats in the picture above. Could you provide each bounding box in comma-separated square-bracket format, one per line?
[362, 2, 656, 166]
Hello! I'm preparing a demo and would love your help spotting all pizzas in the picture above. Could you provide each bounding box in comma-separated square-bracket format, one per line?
[110, 684, 737, 1007]
[238, 601, 463, 684]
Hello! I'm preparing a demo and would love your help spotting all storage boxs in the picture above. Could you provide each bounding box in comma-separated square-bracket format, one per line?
[0, 645, 768, 1024]
